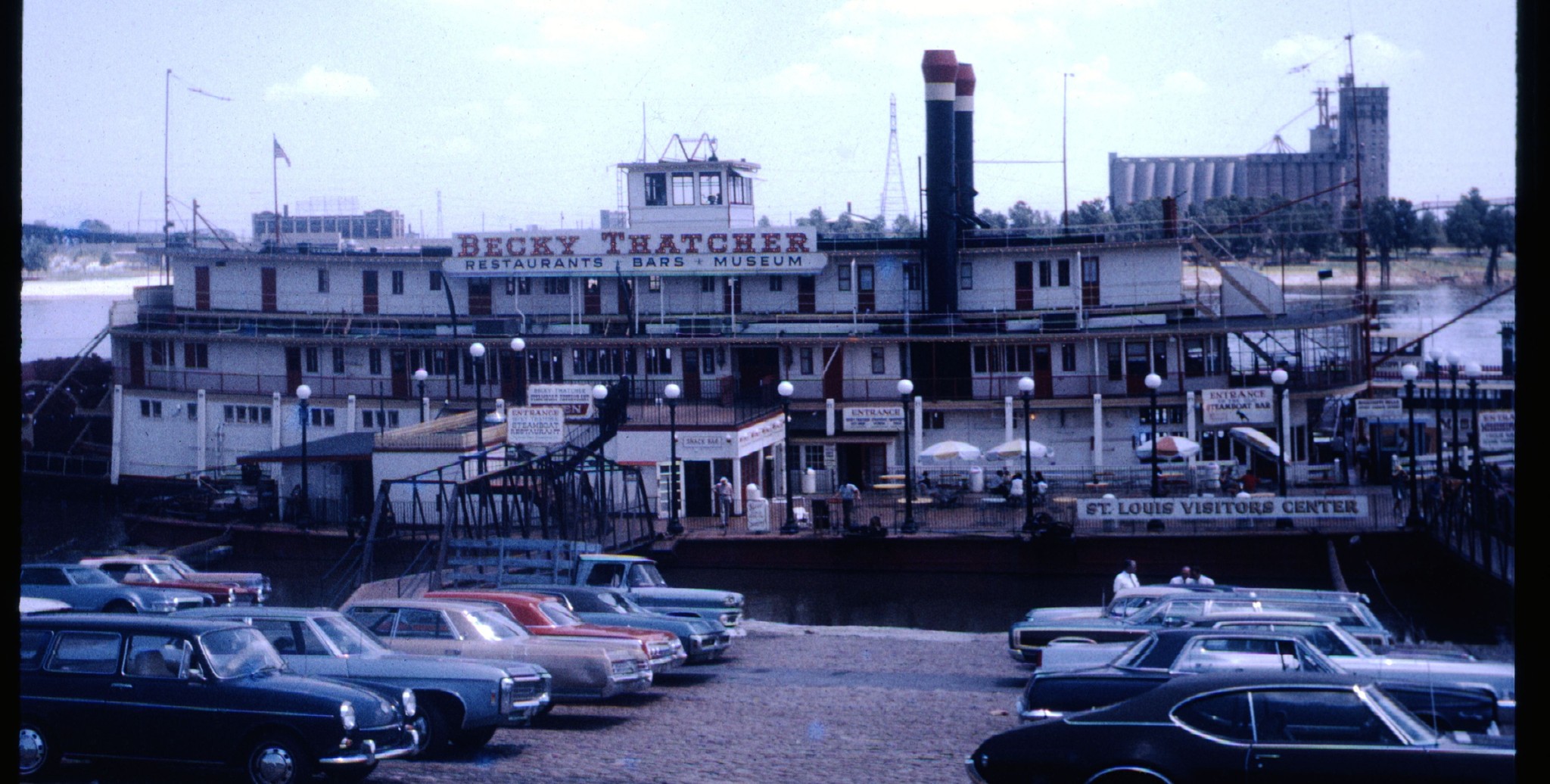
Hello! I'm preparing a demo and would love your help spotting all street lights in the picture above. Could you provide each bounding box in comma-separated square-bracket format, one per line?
[1017, 375, 1038, 531]
[1144, 373, 1162, 499]
[1270, 368, 1292, 496]
[665, 382, 686, 535]
[1402, 362, 1427, 528]
[470, 340, 488, 477]
[898, 377, 920, 536]
[293, 385, 314, 528]
[414, 368, 429, 431]
[510, 338, 530, 411]
[777, 382, 802, 534]
[591, 384, 614, 535]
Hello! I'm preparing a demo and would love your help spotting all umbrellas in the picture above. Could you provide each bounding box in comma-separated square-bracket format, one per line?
[1227, 426, 1292, 486]
[984, 438, 1055, 495]
[1135, 435, 1202, 500]
[916, 439, 980, 487]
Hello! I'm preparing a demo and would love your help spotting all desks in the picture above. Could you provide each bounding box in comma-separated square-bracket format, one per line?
[981, 498, 1006, 526]
[874, 484, 906, 506]
[1054, 497, 1076, 522]
[880, 475, 906, 493]
[1158, 472, 1186, 494]
[1086, 481, 1108, 499]
[893, 496, 935, 531]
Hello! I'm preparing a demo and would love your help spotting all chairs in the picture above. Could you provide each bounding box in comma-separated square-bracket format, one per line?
[135, 649, 176, 678]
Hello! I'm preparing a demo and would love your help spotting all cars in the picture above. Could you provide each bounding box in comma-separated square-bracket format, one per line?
[961, 585, 1517, 784]
[20, 552, 744, 784]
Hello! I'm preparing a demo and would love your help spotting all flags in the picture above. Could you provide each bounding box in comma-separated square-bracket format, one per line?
[1287, 42, 1343, 76]
[274, 140, 293, 166]
[169, 70, 232, 103]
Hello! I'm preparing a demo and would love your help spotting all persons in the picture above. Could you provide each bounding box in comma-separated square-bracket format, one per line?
[909, 466, 1051, 514]
[712, 476, 734, 531]
[832, 474, 861, 528]
[1184, 567, 1216, 587]
[1168, 565, 1196, 586]
[1113, 559, 1142, 607]
[1148, 464, 1267, 504]
[1344, 424, 1512, 526]
